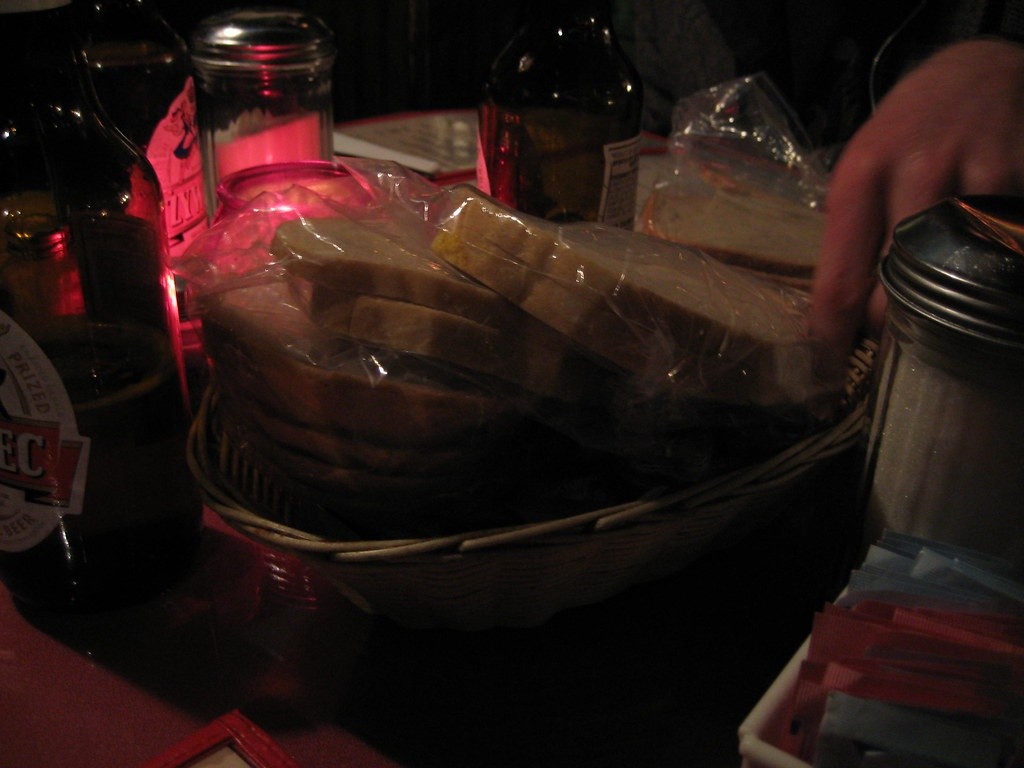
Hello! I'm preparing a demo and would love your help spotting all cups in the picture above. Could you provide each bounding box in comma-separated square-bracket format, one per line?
[218, 156, 358, 213]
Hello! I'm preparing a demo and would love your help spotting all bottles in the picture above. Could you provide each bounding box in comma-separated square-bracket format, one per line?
[77, 0, 222, 377]
[0, 0, 201, 613]
[483, 0, 644, 235]
[196, 16, 337, 210]
[845, 193, 1024, 560]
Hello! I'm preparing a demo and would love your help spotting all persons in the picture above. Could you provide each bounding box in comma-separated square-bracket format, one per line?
[487, 1, 1022, 400]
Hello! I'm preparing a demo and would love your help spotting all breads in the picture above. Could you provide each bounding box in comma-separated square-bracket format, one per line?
[203, 152, 826, 538]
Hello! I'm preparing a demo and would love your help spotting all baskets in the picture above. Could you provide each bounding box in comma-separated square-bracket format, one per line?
[184, 334, 882, 661]
